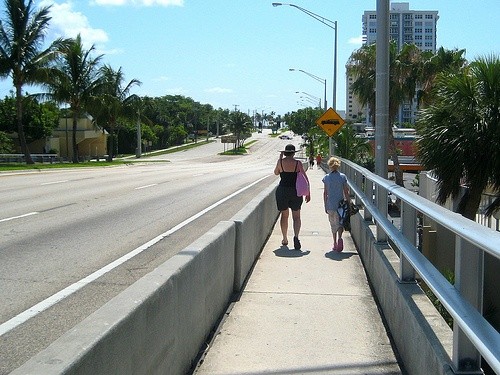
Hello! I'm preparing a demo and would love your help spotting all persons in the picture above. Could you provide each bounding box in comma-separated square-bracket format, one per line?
[316, 154, 323, 167]
[309, 152, 314, 170]
[321, 157, 359, 251]
[273, 143, 310, 250]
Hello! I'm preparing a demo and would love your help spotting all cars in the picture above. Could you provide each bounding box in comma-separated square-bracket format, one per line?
[301, 133, 320, 142]
[279, 134, 294, 140]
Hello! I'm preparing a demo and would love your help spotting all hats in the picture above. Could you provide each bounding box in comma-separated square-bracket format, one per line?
[278, 145, 298, 152]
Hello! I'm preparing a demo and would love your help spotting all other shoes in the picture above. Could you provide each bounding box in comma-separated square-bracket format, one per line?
[333, 244, 338, 250]
[338, 238, 343, 252]
[281, 239, 287, 245]
[294, 237, 301, 250]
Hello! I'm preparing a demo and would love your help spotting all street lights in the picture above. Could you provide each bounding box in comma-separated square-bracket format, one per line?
[272, 2, 338, 156]
[298, 103, 307, 120]
[289, 68, 328, 111]
[295, 91, 322, 152]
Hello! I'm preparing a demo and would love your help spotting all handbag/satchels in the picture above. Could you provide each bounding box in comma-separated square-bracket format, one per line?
[296, 161, 309, 197]
